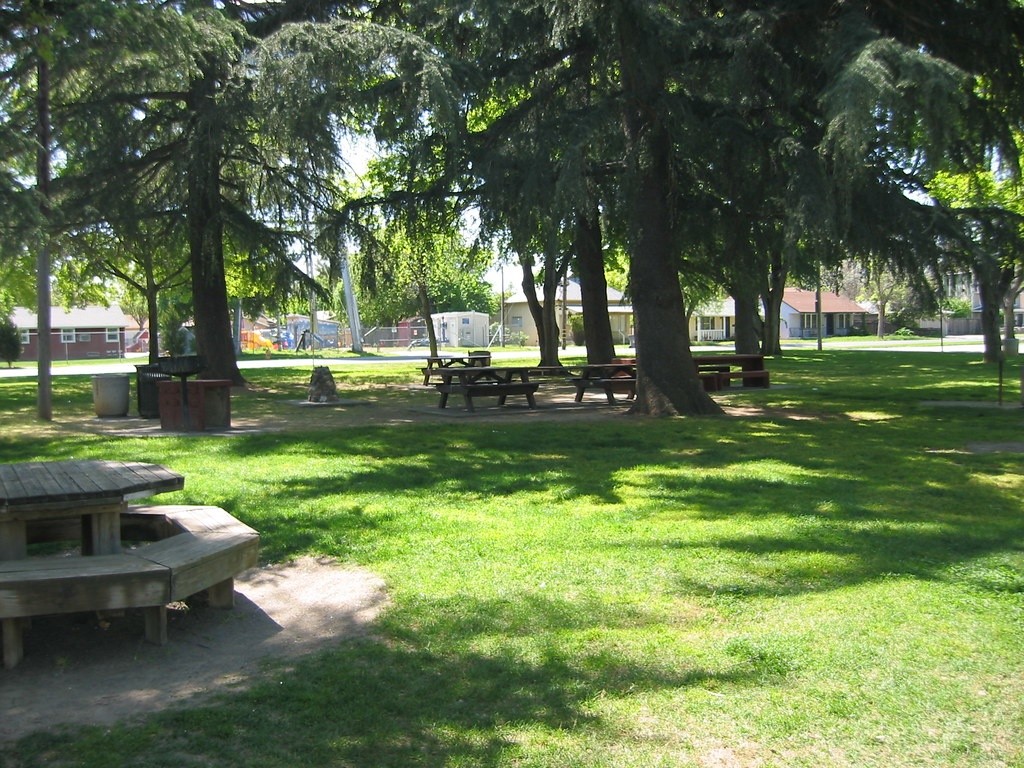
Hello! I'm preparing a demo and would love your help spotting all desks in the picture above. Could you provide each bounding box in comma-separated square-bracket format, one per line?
[423, 366, 538, 413]
[562, 364, 638, 405]
[422, 356, 492, 386]
[0, 460, 186, 630]
[693, 355, 765, 387]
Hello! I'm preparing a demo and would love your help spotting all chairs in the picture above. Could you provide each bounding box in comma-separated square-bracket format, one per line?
[106, 350, 112, 358]
[111, 350, 117, 358]
[118, 350, 123, 358]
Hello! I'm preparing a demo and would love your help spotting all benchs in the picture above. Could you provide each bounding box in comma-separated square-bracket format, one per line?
[564, 376, 631, 382]
[426, 379, 516, 386]
[415, 366, 466, 369]
[698, 371, 769, 391]
[462, 380, 547, 389]
[0, 502, 261, 669]
[699, 366, 730, 388]
[590, 378, 637, 384]
[87, 352, 101, 358]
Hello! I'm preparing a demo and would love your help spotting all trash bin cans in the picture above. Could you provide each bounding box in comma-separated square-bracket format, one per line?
[628, 335, 635, 348]
[134, 364, 172, 419]
[468, 351, 491, 366]
